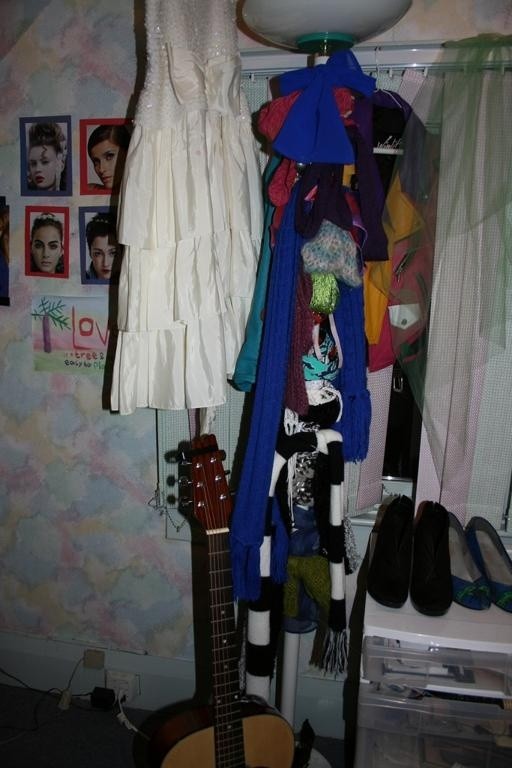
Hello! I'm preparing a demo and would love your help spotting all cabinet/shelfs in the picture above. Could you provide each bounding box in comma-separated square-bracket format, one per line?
[335, 509, 511, 766]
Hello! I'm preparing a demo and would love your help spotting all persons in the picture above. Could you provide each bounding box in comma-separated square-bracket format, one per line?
[25, 120, 67, 193]
[87, 124, 130, 193]
[86, 211, 120, 281]
[28, 212, 64, 274]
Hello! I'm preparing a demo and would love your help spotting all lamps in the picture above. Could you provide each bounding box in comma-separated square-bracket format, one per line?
[232, 0, 418, 63]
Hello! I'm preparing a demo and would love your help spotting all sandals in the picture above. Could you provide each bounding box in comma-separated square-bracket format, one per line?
[448, 509, 494, 612]
[412, 501, 453, 617]
[367, 496, 416, 609]
[466, 517, 512, 612]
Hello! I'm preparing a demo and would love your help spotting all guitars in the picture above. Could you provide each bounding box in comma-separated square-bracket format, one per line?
[135, 433, 296, 768]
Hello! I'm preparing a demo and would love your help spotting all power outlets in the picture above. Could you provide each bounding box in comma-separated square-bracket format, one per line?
[106, 671, 134, 703]
